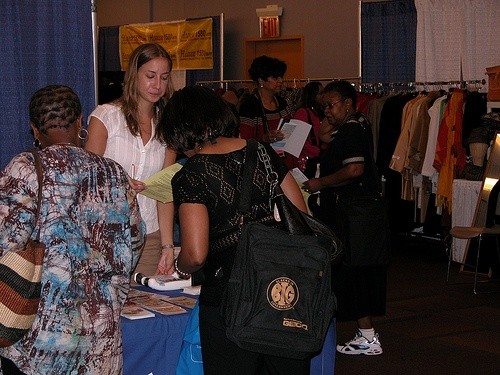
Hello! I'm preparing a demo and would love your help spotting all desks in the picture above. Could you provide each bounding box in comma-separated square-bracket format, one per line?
[452, 179, 483, 264]
[120, 285, 337, 375]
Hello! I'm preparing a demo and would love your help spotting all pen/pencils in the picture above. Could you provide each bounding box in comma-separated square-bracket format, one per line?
[131, 164, 135, 179]
[277, 118, 283, 130]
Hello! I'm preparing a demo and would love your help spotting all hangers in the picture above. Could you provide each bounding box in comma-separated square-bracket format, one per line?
[211, 78, 307, 97]
[362, 81, 478, 98]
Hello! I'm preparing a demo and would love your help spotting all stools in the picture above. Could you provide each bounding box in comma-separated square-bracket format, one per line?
[447, 226, 500, 295]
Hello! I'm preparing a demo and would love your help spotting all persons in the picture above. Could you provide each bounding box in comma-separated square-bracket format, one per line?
[1, 84, 147, 374]
[84, 42, 383, 357]
[157, 84, 308, 374]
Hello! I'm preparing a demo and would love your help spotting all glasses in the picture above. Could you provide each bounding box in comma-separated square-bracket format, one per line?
[324, 98, 347, 108]
[316, 90, 324, 96]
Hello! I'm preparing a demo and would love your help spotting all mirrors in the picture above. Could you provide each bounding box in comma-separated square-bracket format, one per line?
[459, 131, 500, 279]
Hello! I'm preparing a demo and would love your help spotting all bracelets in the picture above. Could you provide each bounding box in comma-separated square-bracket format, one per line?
[161, 244, 174, 249]
[174, 259, 191, 276]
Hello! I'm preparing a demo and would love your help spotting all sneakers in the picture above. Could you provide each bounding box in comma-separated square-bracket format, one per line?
[336, 328, 383, 355]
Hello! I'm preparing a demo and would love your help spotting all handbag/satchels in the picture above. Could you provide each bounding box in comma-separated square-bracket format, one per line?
[0, 150, 46, 348]
[224, 220, 339, 359]
